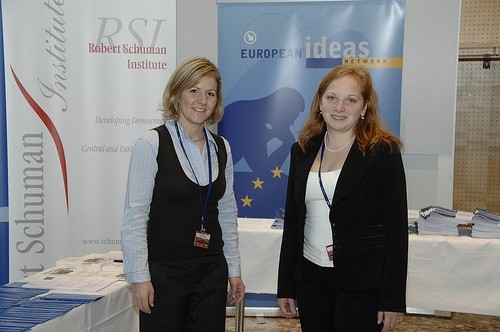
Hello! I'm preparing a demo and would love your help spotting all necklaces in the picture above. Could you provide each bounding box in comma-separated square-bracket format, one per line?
[324, 131, 355, 151]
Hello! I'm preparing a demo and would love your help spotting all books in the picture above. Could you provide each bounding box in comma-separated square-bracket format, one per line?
[408, 207, 500, 239]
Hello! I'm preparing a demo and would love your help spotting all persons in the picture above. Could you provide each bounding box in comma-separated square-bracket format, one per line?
[279, 68, 410, 332]
[121, 57, 245, 332]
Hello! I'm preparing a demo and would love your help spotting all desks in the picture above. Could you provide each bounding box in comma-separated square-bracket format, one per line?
[0, 251, 139, 332]
[226, 218, 500, 332]
